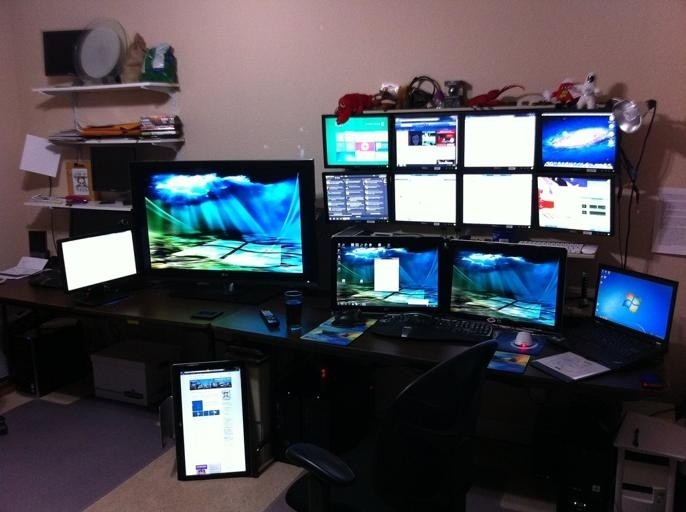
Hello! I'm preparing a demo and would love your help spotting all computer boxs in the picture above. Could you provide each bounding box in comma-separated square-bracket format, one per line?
[613, 402, 678, 512]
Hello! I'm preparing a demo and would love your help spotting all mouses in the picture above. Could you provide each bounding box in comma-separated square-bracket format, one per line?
[582, 244, 598, 255]
[514, 332, 533, 346]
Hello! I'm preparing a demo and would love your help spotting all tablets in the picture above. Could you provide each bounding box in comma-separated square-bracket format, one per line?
[170, 360, 253, 482]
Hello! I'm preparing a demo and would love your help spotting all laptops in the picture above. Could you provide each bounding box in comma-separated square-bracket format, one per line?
[545, 262, 679, 375]
[55, 227, 154, 307]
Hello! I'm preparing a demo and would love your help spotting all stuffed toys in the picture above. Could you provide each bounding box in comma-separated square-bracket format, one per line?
[569, 74, 601, 110]
[546, 78, 576, 109]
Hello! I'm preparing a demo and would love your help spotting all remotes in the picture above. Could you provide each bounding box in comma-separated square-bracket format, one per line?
[258, 307, 282, 330]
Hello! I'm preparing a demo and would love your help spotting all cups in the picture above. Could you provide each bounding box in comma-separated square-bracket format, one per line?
[284, 290, 303, 332]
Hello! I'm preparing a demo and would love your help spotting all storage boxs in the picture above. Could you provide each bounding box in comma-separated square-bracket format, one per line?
[85, 340, 173, 408]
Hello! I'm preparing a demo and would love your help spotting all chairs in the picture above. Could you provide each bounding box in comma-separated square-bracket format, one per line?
[289, 340, 501, 512]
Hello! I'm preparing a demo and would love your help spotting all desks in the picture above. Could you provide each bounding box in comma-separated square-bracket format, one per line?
[1, 261, 667, 506]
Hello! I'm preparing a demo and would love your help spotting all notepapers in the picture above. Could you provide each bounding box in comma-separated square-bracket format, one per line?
[19, 133, 64, 178]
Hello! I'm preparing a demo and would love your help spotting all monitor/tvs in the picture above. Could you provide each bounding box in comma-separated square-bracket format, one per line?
[321, 113, 392, 171]
[129, 159, 320, 306]
[459, 171, 535, 230]
[390, 172, 459, 228]
[460, 107, 539, 171]
[438, 238, 569, 335]
[391, 110, 462, 173]
[321, 171, 392, 225]
[535, 172, 616, 238]
[41, 28, 95, 86]
[89, 145, 177, 205]
[539, 107, 621, 176]
[324, 235, 448, 318]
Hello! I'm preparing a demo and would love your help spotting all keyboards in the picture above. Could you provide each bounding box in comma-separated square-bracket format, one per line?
[518, 240, 585, 254]
[367, 313, 495, 346]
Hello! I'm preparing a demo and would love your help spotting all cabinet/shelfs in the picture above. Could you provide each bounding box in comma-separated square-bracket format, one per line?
[614, 411, 686, 511]
